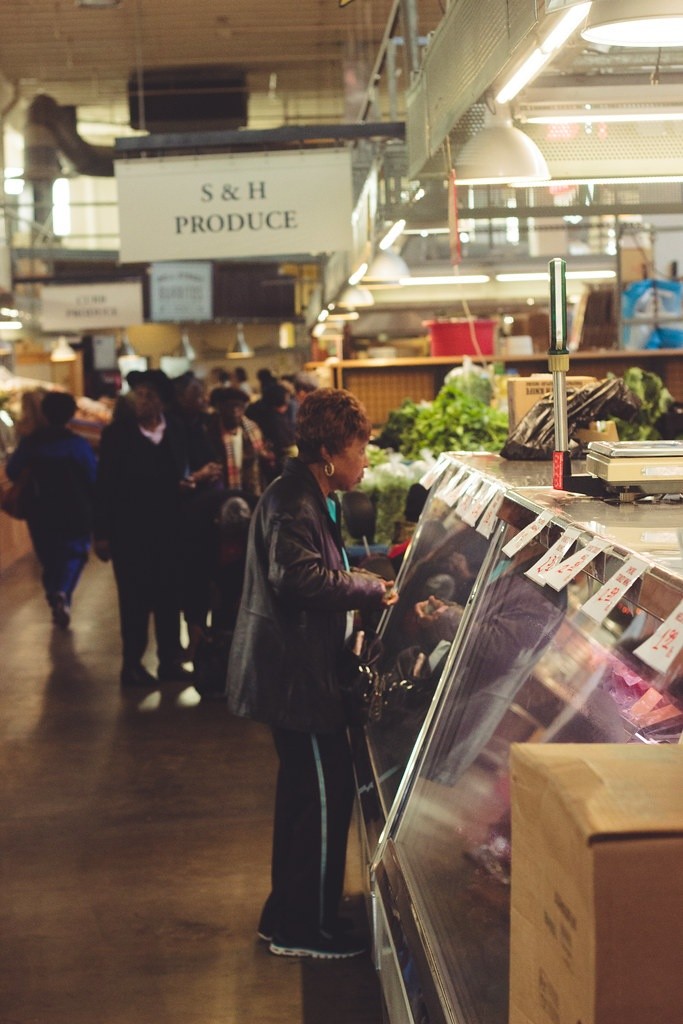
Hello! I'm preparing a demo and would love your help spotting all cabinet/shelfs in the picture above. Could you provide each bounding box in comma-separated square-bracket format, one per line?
[345, 452, 683, 1024]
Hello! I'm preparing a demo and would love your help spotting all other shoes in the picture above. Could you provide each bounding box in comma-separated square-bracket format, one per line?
[158, 662, 194, 682]
[121, 664, 160, 687]
[47, 590, 70, 625]
[258, 891, 369, 959]
[197, 686, 228, 702]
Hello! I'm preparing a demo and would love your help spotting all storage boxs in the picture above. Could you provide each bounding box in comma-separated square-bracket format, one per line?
[506, 742, 683, 1024]
[510, 375, 621, 443]
[425, 318, 500, 357]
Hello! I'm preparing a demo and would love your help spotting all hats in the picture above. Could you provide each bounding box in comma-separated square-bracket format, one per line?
[127, 369, 176, 404]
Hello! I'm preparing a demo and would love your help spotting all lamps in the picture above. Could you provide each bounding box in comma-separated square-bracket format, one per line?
[447, 0, 683, 186]
[50, 334, 79, 363]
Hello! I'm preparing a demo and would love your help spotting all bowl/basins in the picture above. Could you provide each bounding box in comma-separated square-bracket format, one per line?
[421, 320, 497, 358]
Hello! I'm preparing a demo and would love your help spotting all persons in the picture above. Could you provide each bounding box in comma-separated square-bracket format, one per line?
[92, 366, 319, 686]
[414, 527, 569, 785]
[225, 388, 399, 960]
[0, 388, 97, 627]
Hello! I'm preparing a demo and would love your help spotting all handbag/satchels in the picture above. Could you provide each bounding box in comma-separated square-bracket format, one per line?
[341, 645, 379, 730]
[5, 439, 42, 519]
